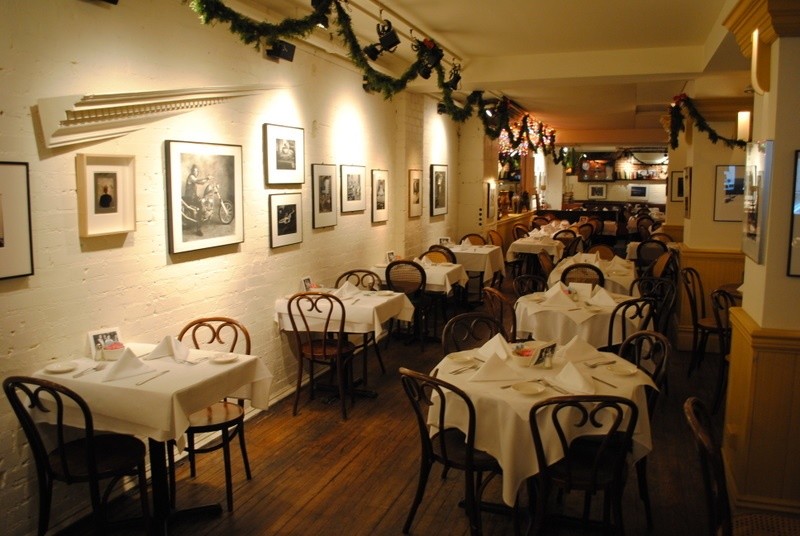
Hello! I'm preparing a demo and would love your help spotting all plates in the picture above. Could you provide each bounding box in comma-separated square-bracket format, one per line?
[612, 270, 628, 276]
[608, 364, 638, 376]
[511, 350, 535, 359]
[526, 296, 542, 301]
[376, 264, 387, 268]
[285, 295, 295, 298]
[45, 361, 78, 372]
[211, 353, 238, 362]
[447, 351, 473, 361]
[440, 262, 453, 266]
[377, 290, 393, 295]
[585, 305, 602, 311]
[512, 382, 544, 394]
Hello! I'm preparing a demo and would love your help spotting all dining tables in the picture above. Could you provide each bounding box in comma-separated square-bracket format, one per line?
[549, 253, 640, 297]
[28, 341, 275, 536]
[427, 340, 660, 536]
[274, 290, 415, 424]
[374, 263, 469, 346]
[513, 285, 656, 366]
[445, 244, 504, 307]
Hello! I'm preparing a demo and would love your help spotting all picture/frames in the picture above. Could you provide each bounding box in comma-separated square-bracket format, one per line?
[0, 161, 34, 280]
[408, 164, 448, 217]
[671, 142, 800, 277]
[588, 185, 606, 198]
[264, 120, 389, 249]
[165, 140, 244, 254]
[77, 152, 136, 239]
[628, 184, 648, 200]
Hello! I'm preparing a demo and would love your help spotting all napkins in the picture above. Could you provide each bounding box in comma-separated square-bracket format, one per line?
[470, 353, 519, 380]
[557, 361, 591, 392]
[105, 346, 147, 381]
[560, 336, 598, 358]
[481, 333, 511, 359]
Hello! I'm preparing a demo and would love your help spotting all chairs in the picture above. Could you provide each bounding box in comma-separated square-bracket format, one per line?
[0, 215, 744, 536]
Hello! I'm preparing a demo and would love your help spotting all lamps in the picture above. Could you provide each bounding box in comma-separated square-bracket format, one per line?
[312, 0, 498, 119]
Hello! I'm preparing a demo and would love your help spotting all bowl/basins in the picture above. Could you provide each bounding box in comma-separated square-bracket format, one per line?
[103, 347, 125, 361]
